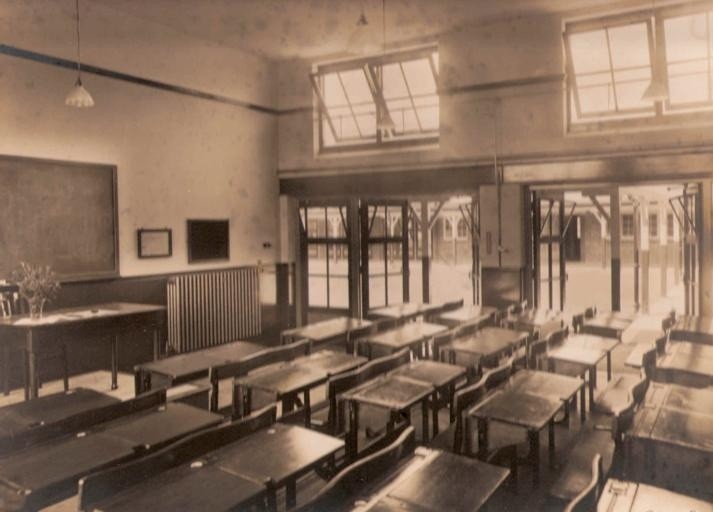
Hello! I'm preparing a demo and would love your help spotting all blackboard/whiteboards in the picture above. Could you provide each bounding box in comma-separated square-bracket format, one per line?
[187, 219, 230, 264]
[0, 155, 119, 287]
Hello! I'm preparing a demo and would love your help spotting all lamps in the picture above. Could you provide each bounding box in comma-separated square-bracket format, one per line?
[639, 0, 669, 101]
[371, 0, 395, 130]
[65, 0, 94, 108]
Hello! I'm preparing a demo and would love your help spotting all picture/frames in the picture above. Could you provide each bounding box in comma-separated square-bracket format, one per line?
[138, 228, 172, 258]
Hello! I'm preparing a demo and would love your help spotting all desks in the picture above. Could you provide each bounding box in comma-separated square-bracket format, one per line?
[0, 301, 713, 512]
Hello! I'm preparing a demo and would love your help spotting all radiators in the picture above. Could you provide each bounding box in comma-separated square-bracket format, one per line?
[166, 267, 262, 350]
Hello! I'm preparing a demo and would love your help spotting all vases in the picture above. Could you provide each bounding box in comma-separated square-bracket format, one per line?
[24, 296, 47, 319]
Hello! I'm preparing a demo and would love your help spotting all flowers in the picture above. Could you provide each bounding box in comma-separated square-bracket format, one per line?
[8, 261, 62, 298]
